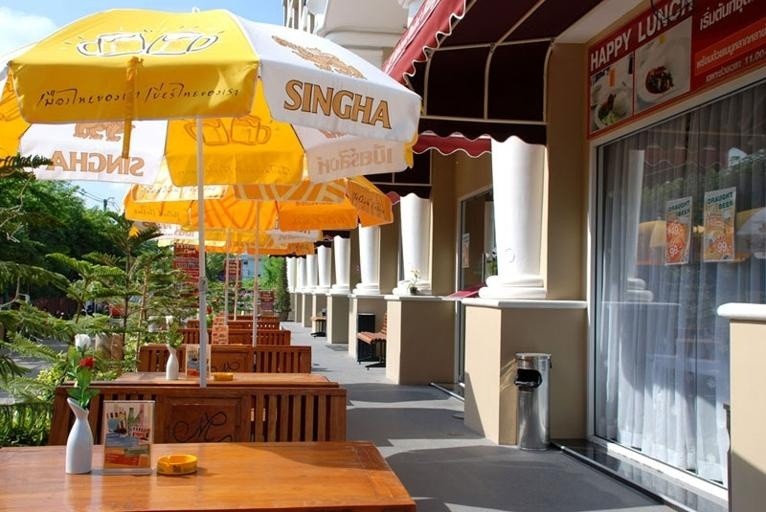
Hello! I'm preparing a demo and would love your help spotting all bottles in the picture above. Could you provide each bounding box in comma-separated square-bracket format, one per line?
[106, 407, 145, 437]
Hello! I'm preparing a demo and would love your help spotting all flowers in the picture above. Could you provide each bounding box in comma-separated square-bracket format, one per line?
[66, 358, 101, 406]
[406, 269, 423, 290]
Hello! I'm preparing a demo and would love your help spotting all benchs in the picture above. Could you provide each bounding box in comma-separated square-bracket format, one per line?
[49, 372, 346, 446]
[136, 314, 312, 371]
[312, 311, 327, 337]
[358, 313, 388, 367]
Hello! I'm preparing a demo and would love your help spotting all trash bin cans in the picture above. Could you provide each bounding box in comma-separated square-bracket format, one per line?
[514, 352, 552, 452]
[321, 308, 326, 332]
[357, 312, 377, 363]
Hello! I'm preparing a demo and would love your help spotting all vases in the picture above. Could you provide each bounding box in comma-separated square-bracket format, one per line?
[65, 396, 93, 474]
[410, 287, 417, 294]
[165, 344, 179, 379]
